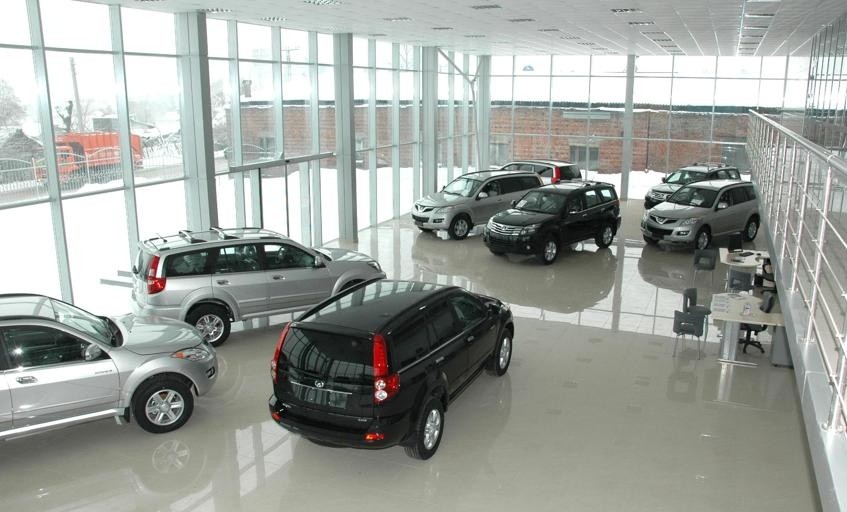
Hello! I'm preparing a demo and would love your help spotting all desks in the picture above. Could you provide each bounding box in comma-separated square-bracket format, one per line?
[711, 293, 784, 365]
[719, 248, 769, 293]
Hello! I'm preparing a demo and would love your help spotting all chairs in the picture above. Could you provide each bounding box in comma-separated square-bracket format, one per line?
[726, 265, 757, 292]
[692, 250, 716, 287]
[727, 234, 742, 249]
[682, 287, 711, 344]
[729, 270, 754, 294]
[672, 310, 703, 360]
[739, 292, 773, 353]
[174, 245, 259, 273]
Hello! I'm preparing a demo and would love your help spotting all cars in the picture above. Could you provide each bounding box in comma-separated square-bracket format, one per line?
[411, 159, 622, 266]
[640, 162, 760, 254]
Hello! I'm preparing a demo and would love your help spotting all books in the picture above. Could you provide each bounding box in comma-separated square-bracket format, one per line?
[726, 292, 745, 300]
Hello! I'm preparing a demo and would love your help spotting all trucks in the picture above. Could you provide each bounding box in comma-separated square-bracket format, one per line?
[32, 131, 145, 192]
